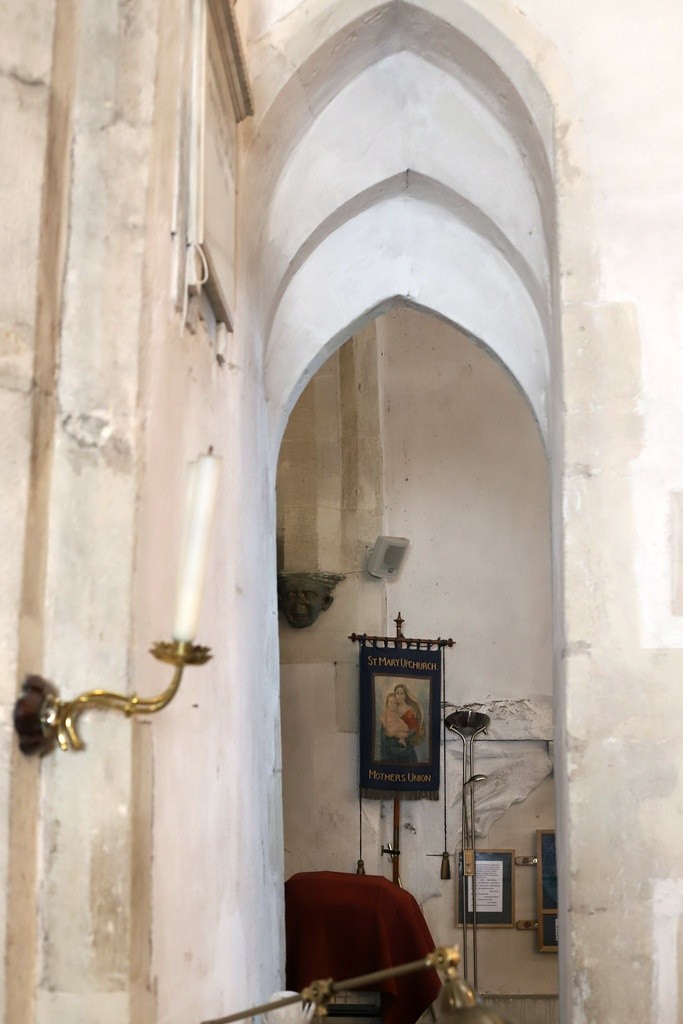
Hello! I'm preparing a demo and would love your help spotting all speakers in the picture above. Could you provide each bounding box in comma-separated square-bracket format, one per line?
[368, 535, 410, 577]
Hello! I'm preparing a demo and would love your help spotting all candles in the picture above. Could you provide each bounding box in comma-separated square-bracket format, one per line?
[172, 445, 218, 642]
